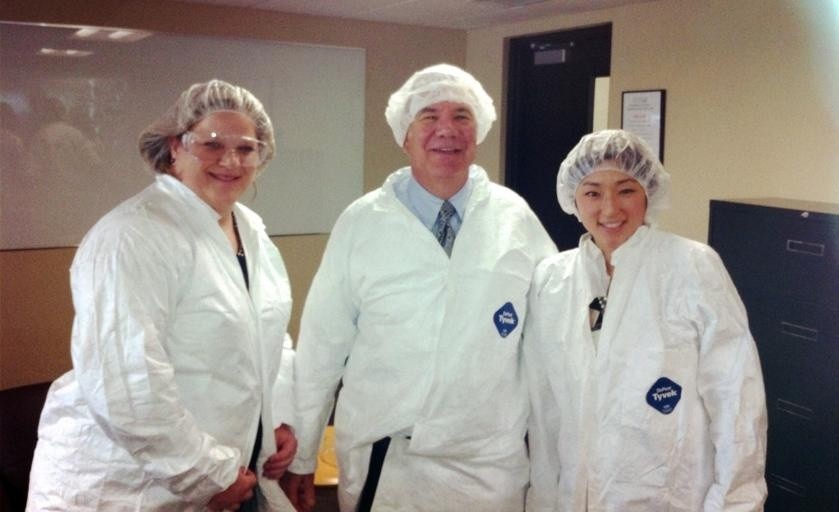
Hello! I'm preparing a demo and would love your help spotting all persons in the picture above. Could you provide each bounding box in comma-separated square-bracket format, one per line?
[282, 60, 560, 511]
[521, 126, 771, 512]
[20, 79, 297, 512]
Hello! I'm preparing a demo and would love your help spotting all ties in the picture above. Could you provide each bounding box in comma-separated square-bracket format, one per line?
[436, 199, 456, 257]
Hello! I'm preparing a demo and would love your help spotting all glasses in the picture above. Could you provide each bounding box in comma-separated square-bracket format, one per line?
[179, 129, 269, 170]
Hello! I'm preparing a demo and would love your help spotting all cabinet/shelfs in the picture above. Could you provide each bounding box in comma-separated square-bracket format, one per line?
[705, 193, 839, 512]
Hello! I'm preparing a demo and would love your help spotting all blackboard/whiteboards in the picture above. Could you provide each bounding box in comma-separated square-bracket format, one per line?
[0, 20, 368, 252]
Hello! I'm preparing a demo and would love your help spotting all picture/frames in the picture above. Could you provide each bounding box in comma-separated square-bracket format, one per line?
[618, 87, 667, 167]
